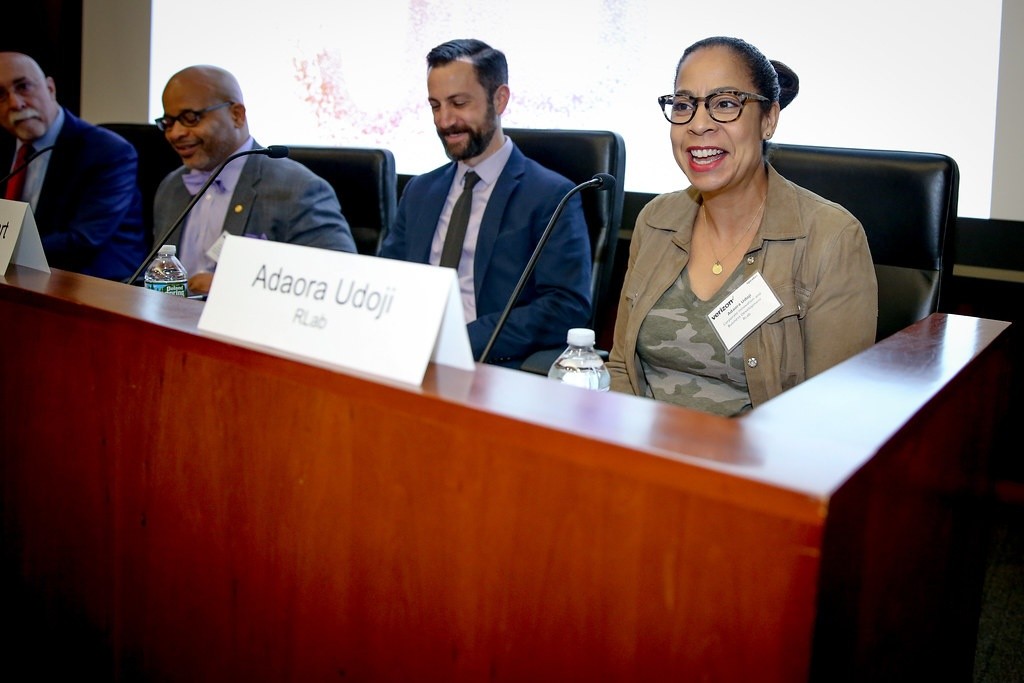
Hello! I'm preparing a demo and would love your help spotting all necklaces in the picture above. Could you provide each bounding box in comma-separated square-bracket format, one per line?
[698, 194, 768, 275]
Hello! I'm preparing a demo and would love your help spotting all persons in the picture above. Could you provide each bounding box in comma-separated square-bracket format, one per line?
[602, 35, 879, 419]
[150, 63, 359, 305]
[0, 51, 147, 285]
[376, 37, 593, 373]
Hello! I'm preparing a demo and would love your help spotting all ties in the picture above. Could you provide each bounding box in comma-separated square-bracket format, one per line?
[440, 171, 480, 269]
[6, 140, 38, 202]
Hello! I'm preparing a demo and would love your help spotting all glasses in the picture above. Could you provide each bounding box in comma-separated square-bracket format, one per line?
[657, 90, 771, 126]
[155, 102, 234, 132]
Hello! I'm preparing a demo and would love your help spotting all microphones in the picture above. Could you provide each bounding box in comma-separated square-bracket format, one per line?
[0, 137, 85, 185]
[126, 145, 289, 286]
[478, 173, 615, 364]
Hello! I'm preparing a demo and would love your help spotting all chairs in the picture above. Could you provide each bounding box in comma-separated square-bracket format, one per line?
[766, 141, 959, 344]
[288, 146, 398, 258]
[98, 122, 183, 262]
[503, 127, 626, 348]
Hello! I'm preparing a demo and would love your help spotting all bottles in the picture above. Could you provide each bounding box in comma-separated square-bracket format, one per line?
[542, 326, 611, 392]
[143, 244, 189, 298]
